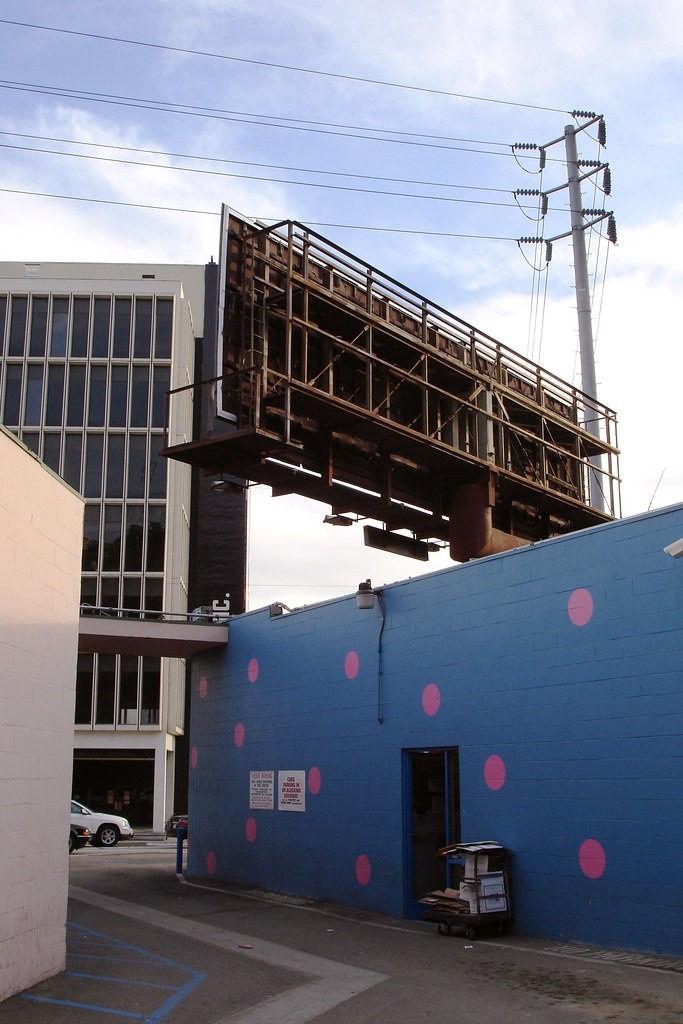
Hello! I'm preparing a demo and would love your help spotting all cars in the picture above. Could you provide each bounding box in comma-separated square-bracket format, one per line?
[165, 815, 188, 837]
[68, 823, 94, 856]
[71, 800, 136, 847]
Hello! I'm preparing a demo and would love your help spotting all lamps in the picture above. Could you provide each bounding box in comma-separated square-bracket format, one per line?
[355, 578, 381, 610]
